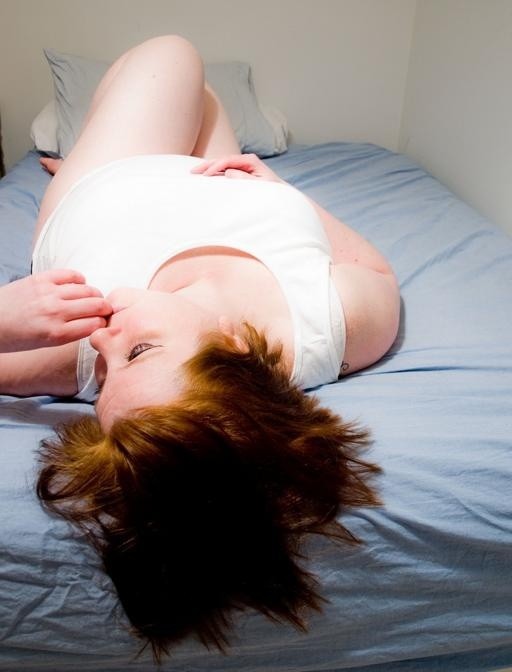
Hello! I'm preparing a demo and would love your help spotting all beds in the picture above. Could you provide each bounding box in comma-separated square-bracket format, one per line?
[0, 141, 511, 670]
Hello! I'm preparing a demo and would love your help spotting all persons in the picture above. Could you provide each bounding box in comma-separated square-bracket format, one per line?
[2, 31, 406, 666]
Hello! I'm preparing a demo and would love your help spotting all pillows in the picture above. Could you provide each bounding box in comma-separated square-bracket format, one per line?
[30, 36, 278, 158]
[30, 96, 288, 159]
